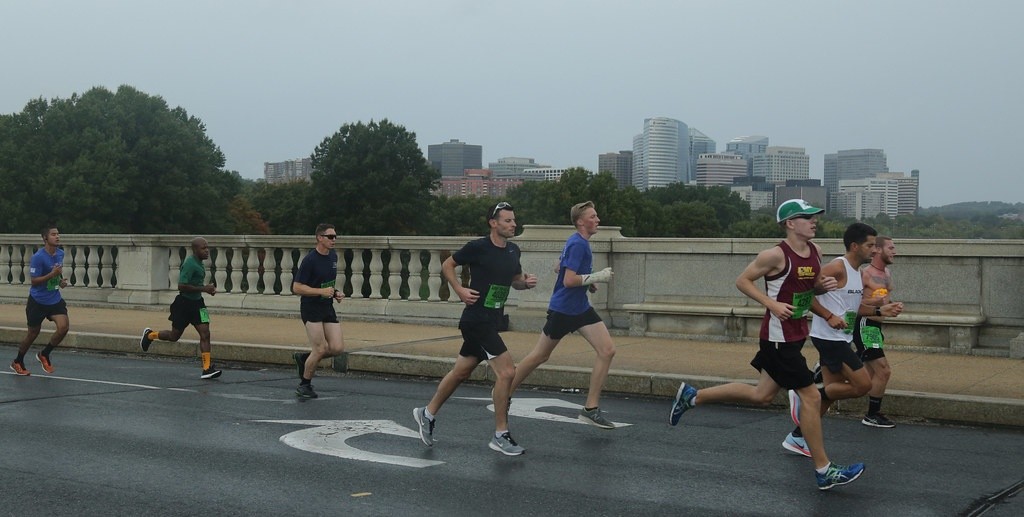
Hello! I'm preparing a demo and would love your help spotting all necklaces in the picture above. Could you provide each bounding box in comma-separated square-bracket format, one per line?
[869, 263, 885, 272]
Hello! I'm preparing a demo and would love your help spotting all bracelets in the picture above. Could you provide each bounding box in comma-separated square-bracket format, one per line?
[876, 306, 882, 315]
[827, 313, 833, 322]
[525, 281, 530, 290]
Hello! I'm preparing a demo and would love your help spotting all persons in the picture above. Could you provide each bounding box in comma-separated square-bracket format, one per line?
[669, 198, 865, 490]
[295, 223, 345, 398]
[10, 223, 70, 376]
[140, 237, 222, 379]
[491, 200, 616, 429]
[414, 202, 537, 456]
[781, 223, 904, 457]
[813, 236, 897, 428]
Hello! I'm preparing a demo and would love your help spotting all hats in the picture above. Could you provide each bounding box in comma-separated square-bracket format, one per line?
[777, 199, 825, 223]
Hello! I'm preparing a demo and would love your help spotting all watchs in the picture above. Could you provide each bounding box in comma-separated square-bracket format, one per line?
[334, 289, 340, 297]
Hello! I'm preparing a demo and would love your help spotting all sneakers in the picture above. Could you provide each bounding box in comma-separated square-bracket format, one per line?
[140, 328, 153, 352]
[295, 384, 318, 398]
[36, 352, 54, 373]
[579, 407, 615, 429]
[669, 382, 697, 426]
[815, 461, 865, 489]
[489, 432, 525, 455]
[292, 351, 305, 379]
[861, 413, 896, 427]
[201, 367, 222, 379]
[789, 390, 802, 426]
[413, 407, 436, 446]
[10, 359, 30, 376]
[782, 432, 811, 457]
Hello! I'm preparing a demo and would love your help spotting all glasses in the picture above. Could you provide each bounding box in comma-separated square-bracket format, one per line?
[784, 215, 812, 225]
[493, 202, 511, 217]
[321, 235, 337, 240]
